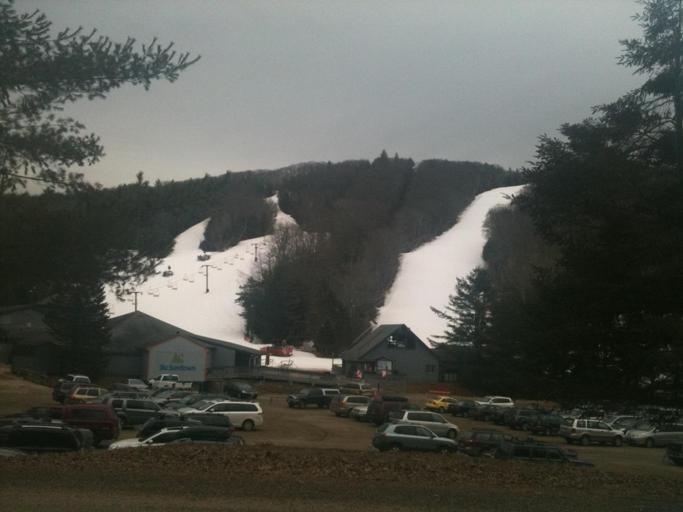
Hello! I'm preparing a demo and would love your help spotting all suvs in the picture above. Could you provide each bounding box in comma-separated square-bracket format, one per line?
[330, 394, 372, 417]
[495, 438, 594, 467]
[108, 426, 246, 449]
[338, 383, 374, 397]
[135, 415, 233, 438]
[392, 408, 459, 439]
[457, 426, 521, 459]
[372, 422, 458, 455]
[368, 395, 424, 426]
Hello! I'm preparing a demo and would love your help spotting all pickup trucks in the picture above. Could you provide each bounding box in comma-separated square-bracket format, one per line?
[286, 387, 341, 410]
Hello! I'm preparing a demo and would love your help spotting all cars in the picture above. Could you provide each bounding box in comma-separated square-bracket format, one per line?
[1, 405, 120, 451]
[320, 371, 336, 382]
[425, 394, 683, 446]
[666, 443, 682, 467]
[350, 404, 368, 420]
[53, 371, 264, 431]
